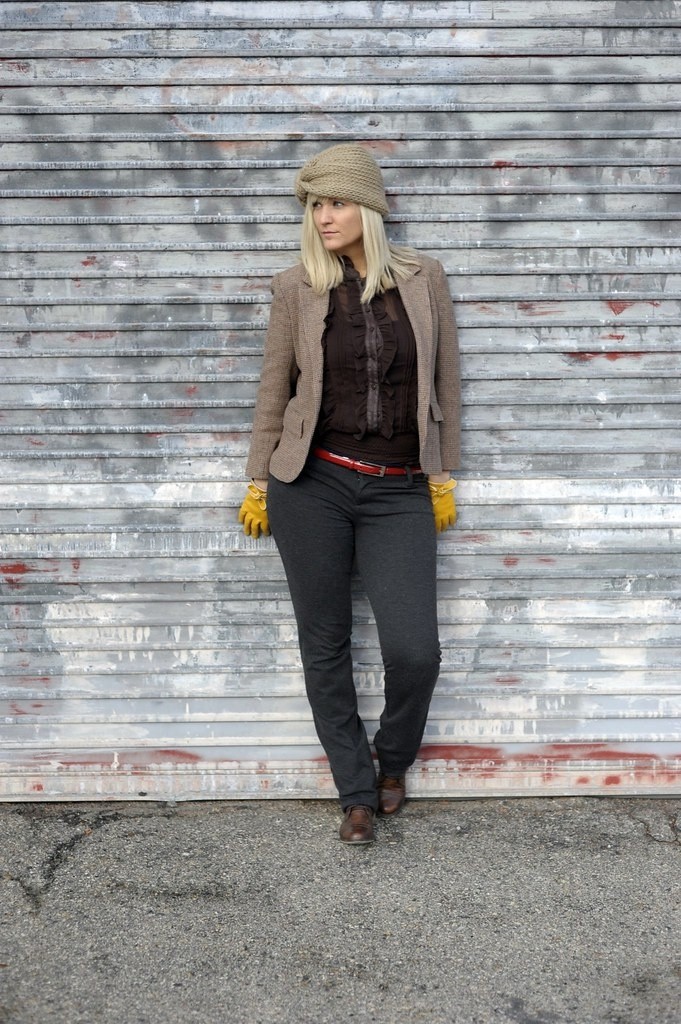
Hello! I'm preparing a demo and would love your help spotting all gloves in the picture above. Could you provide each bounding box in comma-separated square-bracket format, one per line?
[427, 478, 457, 534]
[237, 478, 271, 540]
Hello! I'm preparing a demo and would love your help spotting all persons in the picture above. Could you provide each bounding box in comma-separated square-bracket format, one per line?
[237, 144, 462, 844]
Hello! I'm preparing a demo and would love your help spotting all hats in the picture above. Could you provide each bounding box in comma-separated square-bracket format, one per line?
[294, 143, 390, 219]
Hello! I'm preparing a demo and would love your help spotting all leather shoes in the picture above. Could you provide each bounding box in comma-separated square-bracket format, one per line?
[377, 770, 406, 817]
[339, 804, 375, 845]
[314, 448, 423, 478]
